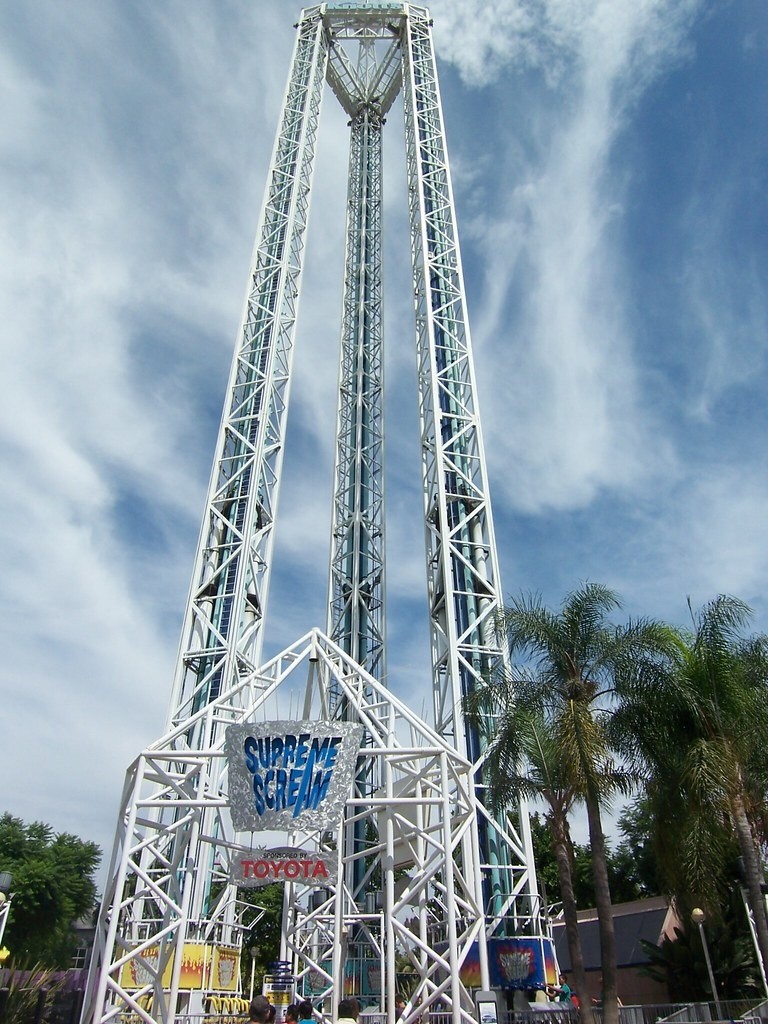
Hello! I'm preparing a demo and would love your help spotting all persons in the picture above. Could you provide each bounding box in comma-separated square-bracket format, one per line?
[543, 975, 579, 1010]
[245, 993, 421, 1024]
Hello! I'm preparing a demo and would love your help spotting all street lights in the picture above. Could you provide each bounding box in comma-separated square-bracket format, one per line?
[691, 907, 724, 1020]
